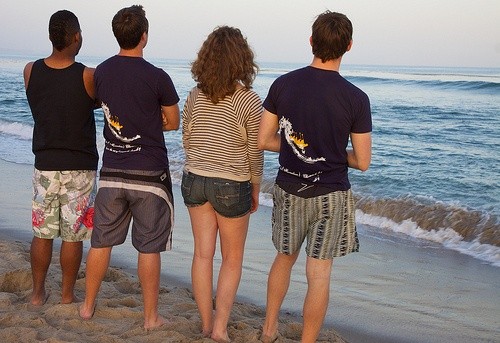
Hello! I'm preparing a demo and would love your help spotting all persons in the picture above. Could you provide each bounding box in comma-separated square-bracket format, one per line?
[258, 13, 372, 343]
[79, 5, 180, 330]
[23, 9, 96, 306]
[181, 25, 264, 343]
